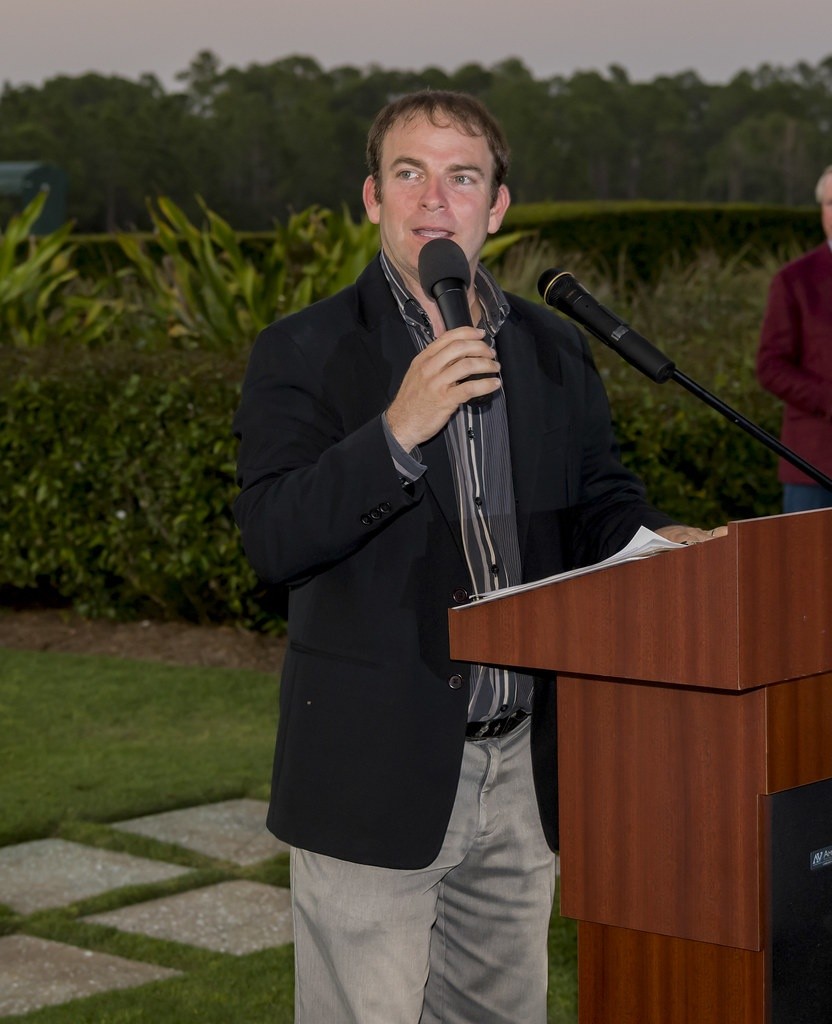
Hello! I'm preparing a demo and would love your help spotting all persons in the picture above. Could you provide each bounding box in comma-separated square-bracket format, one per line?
[226, 89, 726, 1024]
[757, 164, 832, 512]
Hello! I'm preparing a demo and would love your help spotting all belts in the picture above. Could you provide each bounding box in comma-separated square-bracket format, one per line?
[465, 714, 530, 738]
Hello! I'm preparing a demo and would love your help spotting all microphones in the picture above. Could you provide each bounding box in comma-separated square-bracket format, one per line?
[535, 267, 676, 385]
[417, 237, 502, 403]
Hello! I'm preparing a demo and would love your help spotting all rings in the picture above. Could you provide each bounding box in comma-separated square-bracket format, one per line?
[711, 527, 714, 538]
